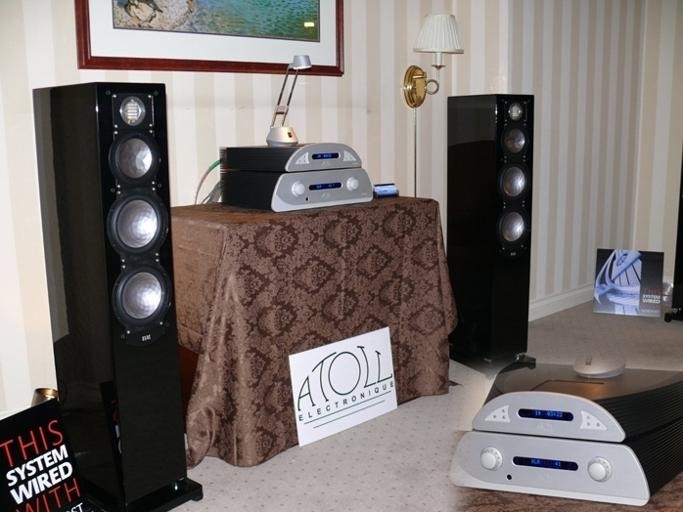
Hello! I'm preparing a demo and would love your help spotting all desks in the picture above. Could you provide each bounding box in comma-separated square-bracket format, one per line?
[171, 194, 457, 468]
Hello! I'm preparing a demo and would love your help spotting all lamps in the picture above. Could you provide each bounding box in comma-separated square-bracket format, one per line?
[403, 14, 464, 108]
[266, 54, 313, 150]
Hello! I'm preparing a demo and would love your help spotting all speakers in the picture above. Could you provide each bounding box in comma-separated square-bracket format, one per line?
[447, 94, 536, 379]
[32, 82, 202, 512]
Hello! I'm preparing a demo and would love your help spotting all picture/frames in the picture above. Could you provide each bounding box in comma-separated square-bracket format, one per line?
[75, 0, 344, 77]
